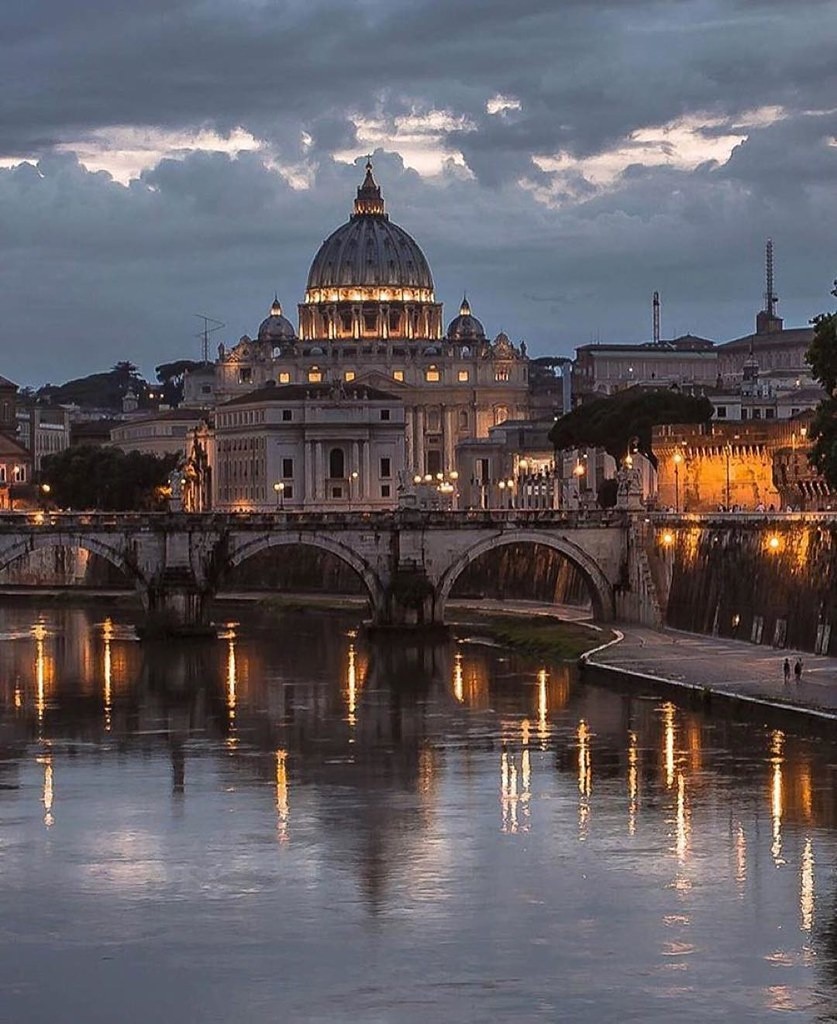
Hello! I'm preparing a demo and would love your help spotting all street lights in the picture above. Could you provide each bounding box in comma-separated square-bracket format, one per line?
[673, 454, 683, 511]
[273, 482, 285, 511]
[348, 472, 358, 510]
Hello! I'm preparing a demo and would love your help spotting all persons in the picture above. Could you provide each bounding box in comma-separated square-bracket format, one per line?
[654, 503, 688, 513]
[715, 502, 800, 513]
[784, 658, 790, 685]
[795, 658, 803, 681]
